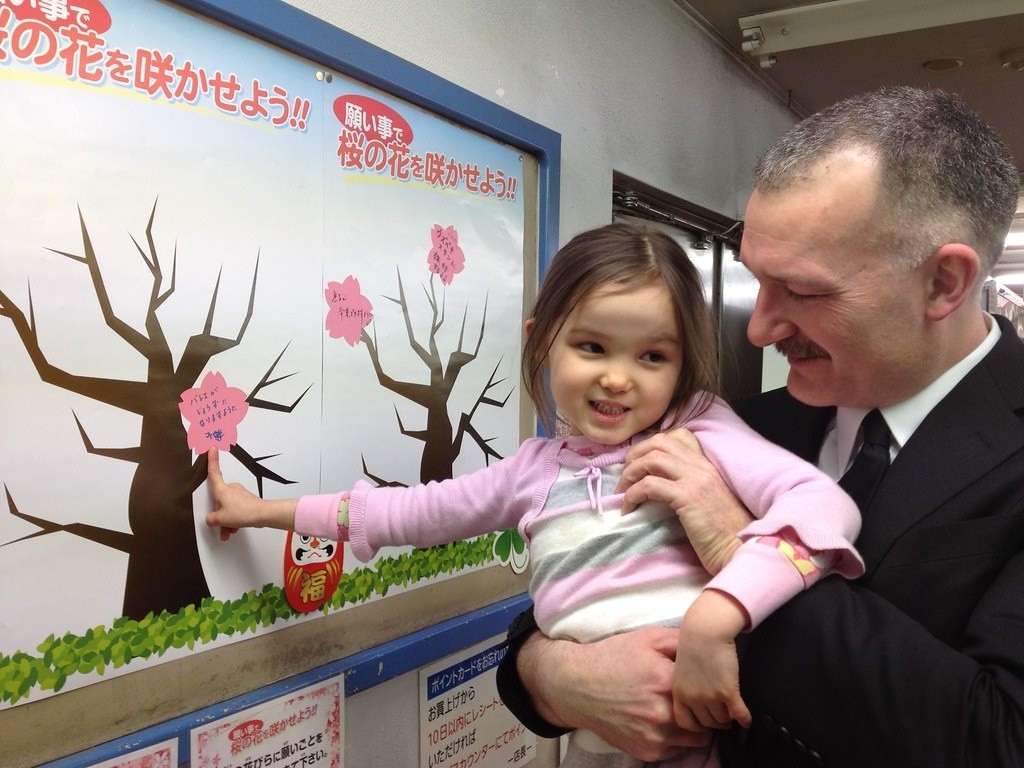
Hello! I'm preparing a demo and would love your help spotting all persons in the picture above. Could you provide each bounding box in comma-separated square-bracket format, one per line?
[206, 226, 865, 768]
[498, 88, 1024, 768]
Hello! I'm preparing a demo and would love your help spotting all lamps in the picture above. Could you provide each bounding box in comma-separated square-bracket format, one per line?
[737, 0, 1024, 71]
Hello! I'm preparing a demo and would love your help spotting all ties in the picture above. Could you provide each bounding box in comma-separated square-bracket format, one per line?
[835, 411, 893, 506]
